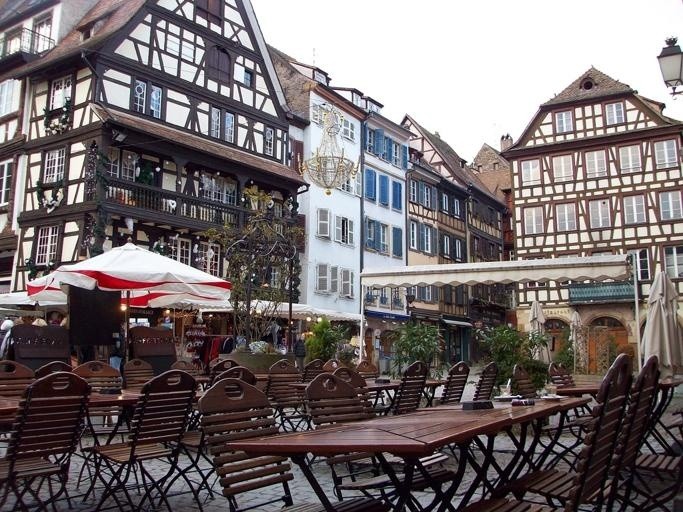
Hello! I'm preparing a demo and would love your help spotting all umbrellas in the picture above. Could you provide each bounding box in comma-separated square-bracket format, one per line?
[568, 311, 588, 367]
[26, 237, 231, 364]
[528, 300, 552, 365]
[640, 270, 683, 382]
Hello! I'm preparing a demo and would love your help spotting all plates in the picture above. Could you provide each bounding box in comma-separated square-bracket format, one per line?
[493, 396, 518, 401]
[540, 396, 563, 401]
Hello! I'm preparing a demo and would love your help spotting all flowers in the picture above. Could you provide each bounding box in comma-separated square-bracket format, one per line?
[40, 95, 71, 135]
[37, 179, 68, 214]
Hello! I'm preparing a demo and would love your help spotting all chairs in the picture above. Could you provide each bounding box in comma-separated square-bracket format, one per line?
[442, 361, 498, 465]
[0, 358, 130, 462]
[415, 361, 470, 465]
[616, 378, 683, 512]
[371, 361, 428, 417]
[508, 354, 661, 511]
[462, 353, 633, 512]
[122, 354, 257, 441]
[325, 367, 450, 474]
[199, 378, 385, 512]
[0, 370, 92, 511]
[511, 361, 600, 438]
[77, 369, 203, 511]
[264, 358, 446, 432]
[304, 372, 457, 511]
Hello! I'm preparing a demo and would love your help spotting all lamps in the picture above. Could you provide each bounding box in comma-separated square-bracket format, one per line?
[298, 102, 359, 200]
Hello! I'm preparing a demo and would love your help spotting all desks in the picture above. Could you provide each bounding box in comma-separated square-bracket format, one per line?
[0, 390, 205, 418]
[226, 396, 594, 511]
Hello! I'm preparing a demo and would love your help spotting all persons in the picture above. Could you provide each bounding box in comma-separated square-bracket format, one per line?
[294, 332, 306, 370]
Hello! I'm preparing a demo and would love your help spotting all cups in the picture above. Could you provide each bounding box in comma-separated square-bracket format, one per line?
[546, 384, 557, 396]
[500, 385, 510, 397]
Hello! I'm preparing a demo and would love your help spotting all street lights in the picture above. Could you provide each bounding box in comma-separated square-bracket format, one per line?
[241, 181, 274, 348]
[283, 196, 299, 355]
